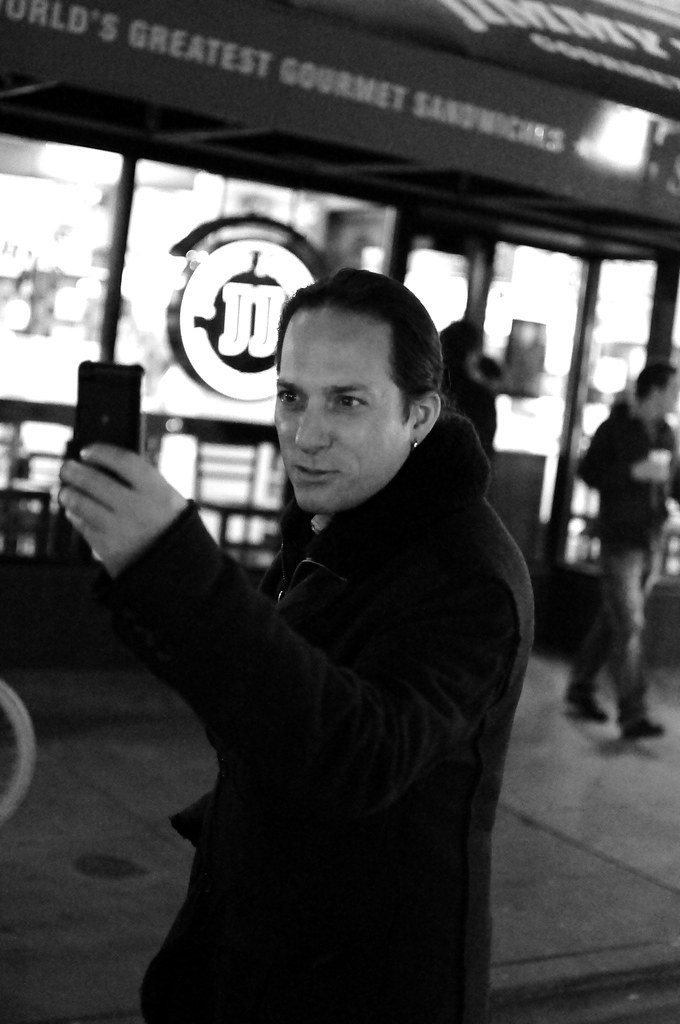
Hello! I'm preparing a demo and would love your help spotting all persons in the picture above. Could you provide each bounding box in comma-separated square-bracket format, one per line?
[440, 318, 499, 462]
[57, 268, 532, 1023]
[564, 363, 680, 740]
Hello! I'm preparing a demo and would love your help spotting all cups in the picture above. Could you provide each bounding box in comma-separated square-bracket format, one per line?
[649, 449, 673, 481]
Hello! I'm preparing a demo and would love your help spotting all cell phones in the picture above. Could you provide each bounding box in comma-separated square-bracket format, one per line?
[76, 358, 144, 498]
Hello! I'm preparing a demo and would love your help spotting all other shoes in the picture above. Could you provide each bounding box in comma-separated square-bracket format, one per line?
[564, 683, 608, 722]
[617, 708, 664, 740]
